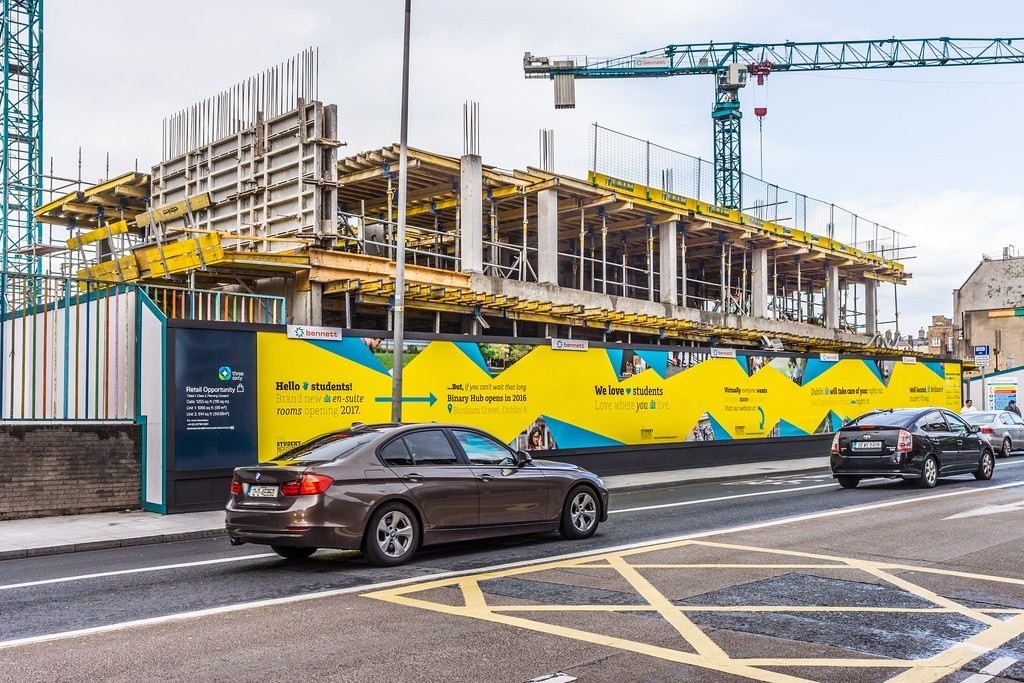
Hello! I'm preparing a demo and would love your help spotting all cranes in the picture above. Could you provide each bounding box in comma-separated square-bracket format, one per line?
[0, 0, 44, 314]
[523, 35, 1024, 210]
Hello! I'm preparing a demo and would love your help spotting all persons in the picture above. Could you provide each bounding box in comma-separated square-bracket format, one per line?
[960, 399, 976, 414]
[730, 293, 739, 313]
[1005, 400, 1021, 417]
[790, 358, 800, 379]
[528, 426, 543, 450]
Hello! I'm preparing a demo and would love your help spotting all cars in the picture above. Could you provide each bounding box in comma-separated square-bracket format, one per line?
[959, 410, 1024, 457]
[830, 407, 996, 488]
[225, 423, 609, 567]
